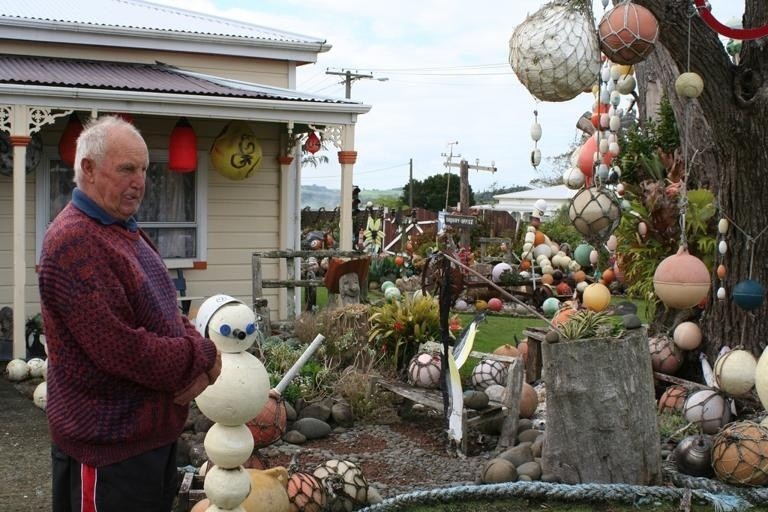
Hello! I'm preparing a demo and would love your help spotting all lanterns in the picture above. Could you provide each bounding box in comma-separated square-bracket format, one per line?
[168, 118, 198, 171]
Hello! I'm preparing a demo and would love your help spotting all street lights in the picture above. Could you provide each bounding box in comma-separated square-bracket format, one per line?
[341, 71, 391, 104]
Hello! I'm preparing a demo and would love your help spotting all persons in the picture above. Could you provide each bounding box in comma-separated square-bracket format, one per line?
[36, 116, 222, 512]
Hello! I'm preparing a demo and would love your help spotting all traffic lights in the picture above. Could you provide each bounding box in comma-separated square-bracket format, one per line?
[351, 185, 361, 218]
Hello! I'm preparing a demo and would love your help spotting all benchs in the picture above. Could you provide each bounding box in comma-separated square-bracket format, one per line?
[364, 339, 525, 454]
